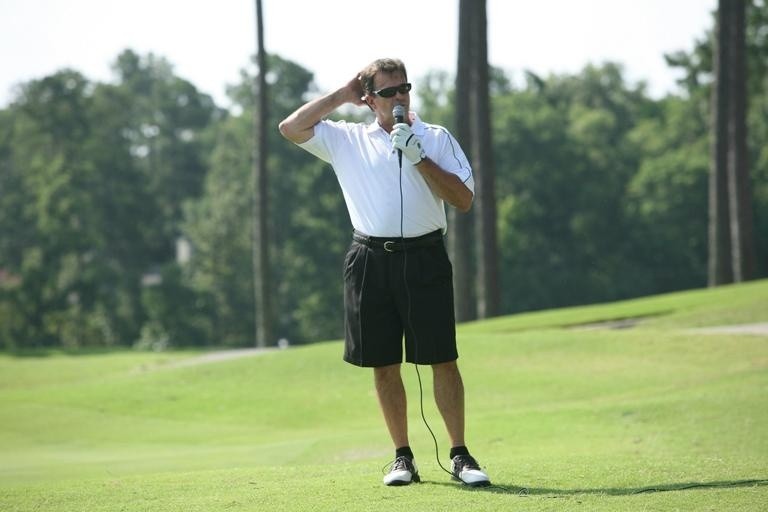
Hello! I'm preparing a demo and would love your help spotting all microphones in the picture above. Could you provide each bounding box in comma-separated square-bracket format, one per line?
[391, 104, 405, 168]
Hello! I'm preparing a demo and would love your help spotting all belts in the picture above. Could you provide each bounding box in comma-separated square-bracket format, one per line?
[352, 233, 440, 252]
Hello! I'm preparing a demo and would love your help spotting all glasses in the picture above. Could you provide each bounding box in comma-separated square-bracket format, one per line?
[371, 83, 411, 98]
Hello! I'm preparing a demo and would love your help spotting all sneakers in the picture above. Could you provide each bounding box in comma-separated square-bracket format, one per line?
[384, 457, 419, 486]
[450, 456, 491, 487]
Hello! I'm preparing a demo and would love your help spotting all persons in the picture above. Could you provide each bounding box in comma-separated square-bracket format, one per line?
[277, 57, 495, 487]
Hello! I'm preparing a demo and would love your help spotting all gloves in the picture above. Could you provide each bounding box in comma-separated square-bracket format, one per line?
[390, 123, 426, 165]
